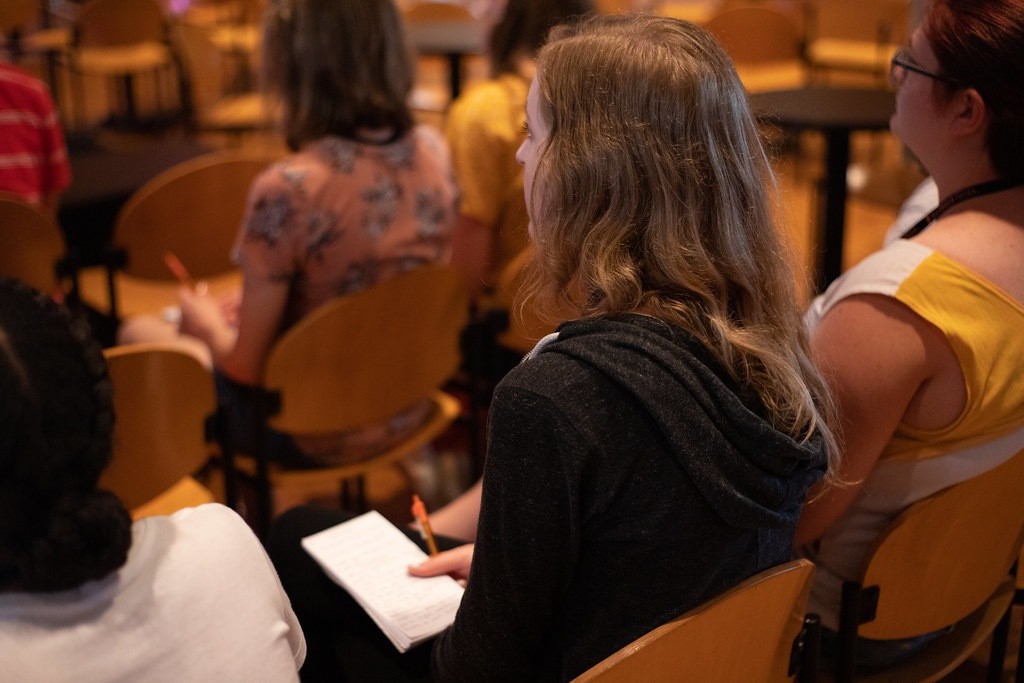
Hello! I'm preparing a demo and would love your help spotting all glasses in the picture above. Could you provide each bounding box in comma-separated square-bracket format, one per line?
[888, 50, 996, 114]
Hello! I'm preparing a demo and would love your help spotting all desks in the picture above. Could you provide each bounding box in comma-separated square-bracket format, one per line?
[748, 88, 896, 295]
[410, 21, 490, 99]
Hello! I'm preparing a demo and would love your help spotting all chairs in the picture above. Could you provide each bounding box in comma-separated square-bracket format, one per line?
[61, 0, 177, 136]
[704, 8, 810, 93]
[567, 559, 815, 683]
[103, 339, 236, 522]
[163, 16, 276, 149]
[0, 0, 74, 98]
[78, 149, 276, 333]
[236, 265, 466, 556]
[815, 450, 1024, 683]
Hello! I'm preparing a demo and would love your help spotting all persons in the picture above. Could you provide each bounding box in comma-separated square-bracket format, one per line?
[118, 0, 460, 465]
[0, 279, 308, 683]
[0, 59, 71, 225]
[445, 0, 606, 377]
[795, 1, 1024, 671]
[266, 15, 847, 683]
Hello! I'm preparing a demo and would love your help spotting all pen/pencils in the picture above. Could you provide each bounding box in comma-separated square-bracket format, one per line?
[166, 254, 197, 294]
[411, 494, 437, 555]
